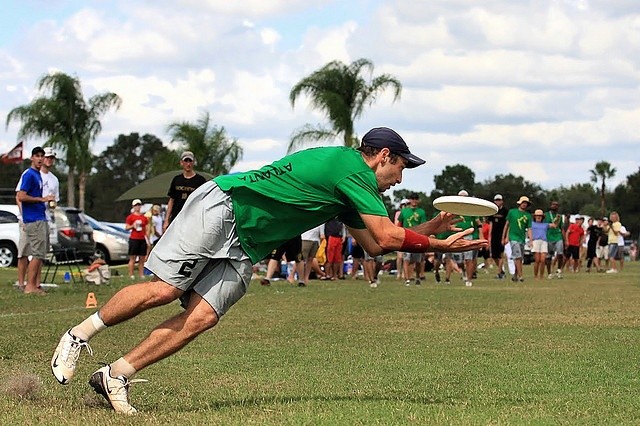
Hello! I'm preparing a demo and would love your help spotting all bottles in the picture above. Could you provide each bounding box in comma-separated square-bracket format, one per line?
[48, 191, 57, 209]
[64, 272, 71, 283]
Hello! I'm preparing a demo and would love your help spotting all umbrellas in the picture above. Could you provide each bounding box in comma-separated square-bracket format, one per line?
[113, 170, 214, 201]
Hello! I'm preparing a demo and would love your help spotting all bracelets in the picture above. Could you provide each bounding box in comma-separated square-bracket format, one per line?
[399, 227, 430, 253]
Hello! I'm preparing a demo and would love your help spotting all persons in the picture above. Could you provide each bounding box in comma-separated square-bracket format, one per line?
[531, 209, 560, 282]
[431, 211, 451, 285]
[563, 215, 574, 231]
[544, 201, 565, 280]
[364, 251, 382, 288]
[586, 217, 606, 273]
[559, 218, 585, 274]
[17, 147, 55, 294]
[596, 218, 610, 273]
[488, 194, 508, 279]
[616, 226, 630, 270]
[163, 151, 206, 233]
[579, 218, 594, 260]
[125, 199, 149, 280]
[15, 147, 60, 292]
[350, 235, 364, 280]
[320, 222, 346, 280]
[501, 196, 534, 282]
[451, 190, 479, 287]
[398, 193, 426, 287]
[606, 211, 622, 273]
[395, 199, 409, 280]
[51, 127, 488, 416]
[479, 216, 490, 274]
[150, 203, 162, 246]
[261, 234, 307, 288]
[286, 223, 319, 285]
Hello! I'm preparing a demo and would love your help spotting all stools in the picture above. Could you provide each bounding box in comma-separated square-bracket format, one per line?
[43, 248, 84, 286]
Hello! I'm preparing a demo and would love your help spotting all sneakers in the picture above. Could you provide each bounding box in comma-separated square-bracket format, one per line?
[50, 328, 92, 385]
[88, 364, 148, 415]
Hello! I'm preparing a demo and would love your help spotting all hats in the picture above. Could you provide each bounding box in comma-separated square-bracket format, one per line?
[180, 151, 195, 162]
[516, 195, 532, 207]
[494, 194, 502, 201]
[459, 190, 468, 195]
[531, 209, 546, 220]
[132, 199, 142, 206]
[32, 146, 45, 155]
[362, 127, 425, 169]
[43, 147, 57, 158]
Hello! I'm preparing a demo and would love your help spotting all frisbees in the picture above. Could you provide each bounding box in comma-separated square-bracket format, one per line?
[433, 196, 498, 216]
